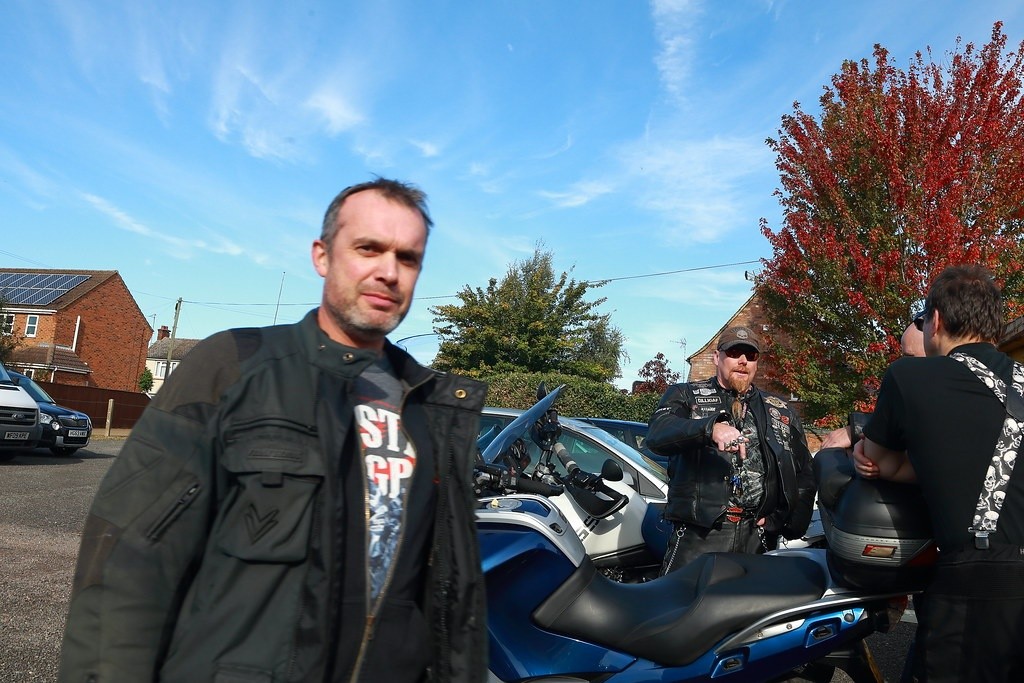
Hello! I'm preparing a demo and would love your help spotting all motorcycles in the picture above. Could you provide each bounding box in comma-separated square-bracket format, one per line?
[476, 381, 937, 683]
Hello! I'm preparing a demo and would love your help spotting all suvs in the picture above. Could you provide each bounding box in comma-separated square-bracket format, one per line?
[1, 361, 39, 464]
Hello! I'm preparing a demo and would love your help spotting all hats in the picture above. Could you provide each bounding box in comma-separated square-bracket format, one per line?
[717, 327, 762, 356]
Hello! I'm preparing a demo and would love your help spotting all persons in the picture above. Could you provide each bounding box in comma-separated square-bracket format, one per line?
[647, 327, 816, 577]
[58, 179, 490, 681]
[820, 265, 1024, 683]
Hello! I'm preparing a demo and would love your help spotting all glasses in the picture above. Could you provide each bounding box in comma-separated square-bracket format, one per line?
[718, 346, 760, 362]
[913, 308, 929, 332]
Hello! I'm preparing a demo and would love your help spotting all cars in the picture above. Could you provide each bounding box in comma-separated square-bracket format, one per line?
[477, 405, 674, 569]
[560, 414, 671, 473]
[5, 369, 93, 456]
